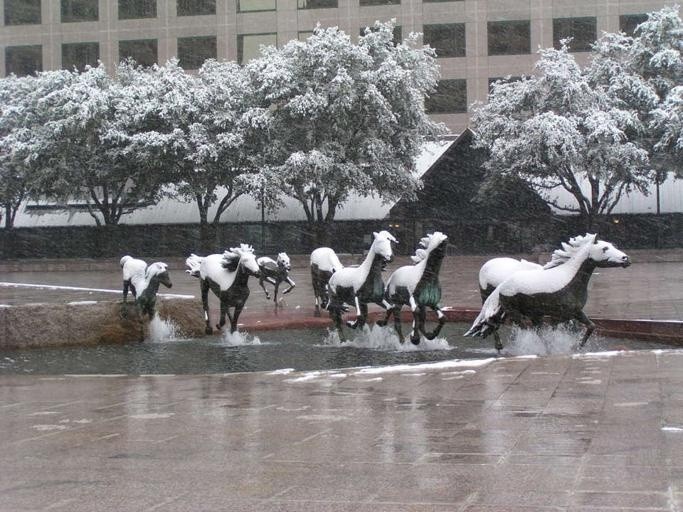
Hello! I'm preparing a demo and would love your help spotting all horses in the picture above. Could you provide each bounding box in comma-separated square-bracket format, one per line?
[185, 243, 263, 335]
[383, 231, 450, 344]
[256, 252, 296, 306]
[327, 230, 394, 343]
[119, 255, 173, 343]
[310, 247, 346, 317]
[462, 233, 632, 354]
